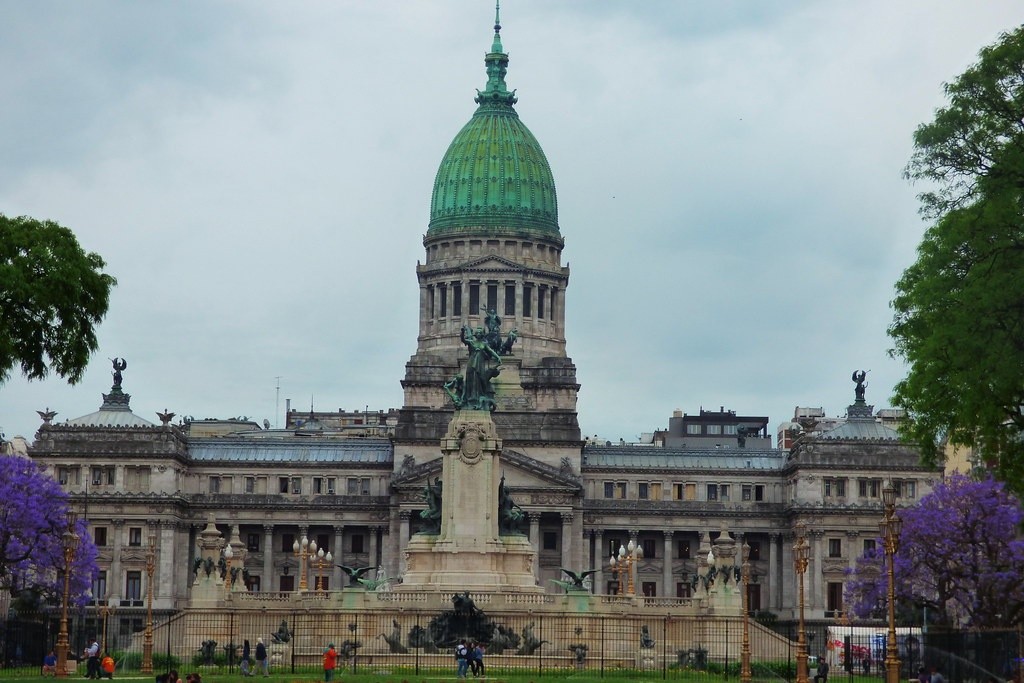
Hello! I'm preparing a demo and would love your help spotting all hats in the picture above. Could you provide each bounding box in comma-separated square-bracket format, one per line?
[328, 641, 335, 647]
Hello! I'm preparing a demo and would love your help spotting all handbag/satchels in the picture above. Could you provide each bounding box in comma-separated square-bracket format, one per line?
[263, 658, 268, 668]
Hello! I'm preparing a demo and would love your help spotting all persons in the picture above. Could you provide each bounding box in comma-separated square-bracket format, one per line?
[240, 638, 269, 678]
[456, 639, 486, 678]
[445, 305, 517, 410]
[192, 557, 250, 586]
[156, 670, 202, 683]
[43, 637, 115, 680]
[424, 476, 443, 532]
[498, 468, 523, 529]
[918, 665, 945, 683]
[814, 657, 828, 683]
[322, 642, 337, 683]
[862, 654, 870, 675]
[270, 619, 290, 643]
[640, 624, 656, 648]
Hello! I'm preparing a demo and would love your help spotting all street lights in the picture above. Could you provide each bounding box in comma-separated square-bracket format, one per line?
[55, 509, 79, 679]
[877, 483, 903, 683]
[141, 532, 158, 675]
[740, 542, 752, 683]
[792, 537, 811, 683]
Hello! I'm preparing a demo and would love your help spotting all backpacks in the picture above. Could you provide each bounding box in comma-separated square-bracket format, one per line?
[470, 648, 479, 660]
